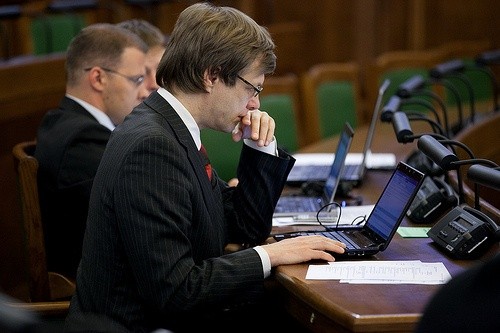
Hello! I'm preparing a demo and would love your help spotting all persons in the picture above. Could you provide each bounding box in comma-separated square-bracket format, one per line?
[33, 19, 167, 287]
[62, 3, 348, 333]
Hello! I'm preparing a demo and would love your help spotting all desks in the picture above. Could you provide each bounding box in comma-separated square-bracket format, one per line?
[223, 93, 500, 333]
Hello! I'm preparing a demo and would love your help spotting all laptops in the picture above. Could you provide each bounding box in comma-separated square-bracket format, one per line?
[274, 122, 355, 217]
[272, 161, 425, 256]
[284, 80, 390, 187]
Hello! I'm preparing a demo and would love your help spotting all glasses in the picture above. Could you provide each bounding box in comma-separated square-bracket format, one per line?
[234, 71, 264, 98]
[84, 67, 145, 87]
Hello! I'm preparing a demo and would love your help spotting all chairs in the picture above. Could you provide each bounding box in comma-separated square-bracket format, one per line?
[0, 0, 500, 314]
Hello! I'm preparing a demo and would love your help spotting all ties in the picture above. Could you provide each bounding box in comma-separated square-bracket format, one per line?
[199, 143, 212, 184]
